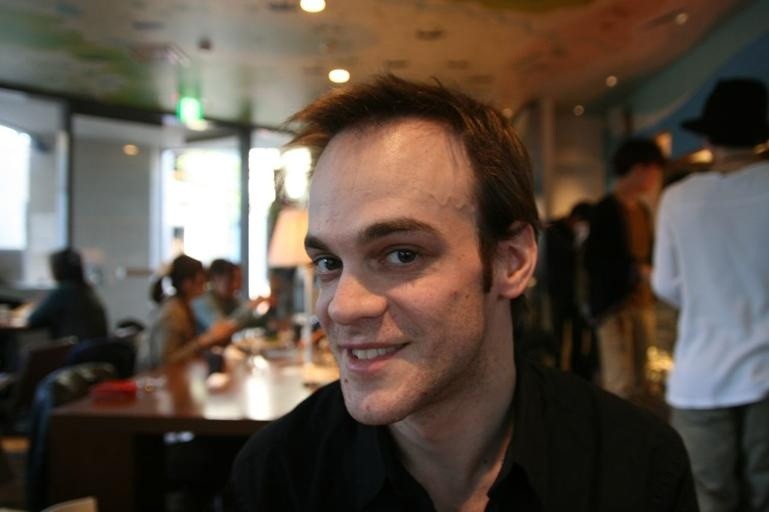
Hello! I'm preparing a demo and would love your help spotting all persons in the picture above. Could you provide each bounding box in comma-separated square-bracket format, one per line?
[225, 74, 697, 512]
[0, 246, 107, 417]
[649, 77, 768, 511]
[542, 135, 667, 399]
[150, 255, 276, 372]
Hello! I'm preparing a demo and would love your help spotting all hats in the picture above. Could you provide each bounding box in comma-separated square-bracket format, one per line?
[679, 77, 765, 148]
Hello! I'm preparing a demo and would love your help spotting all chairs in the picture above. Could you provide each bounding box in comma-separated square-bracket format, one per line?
[9, 326, 140, 422]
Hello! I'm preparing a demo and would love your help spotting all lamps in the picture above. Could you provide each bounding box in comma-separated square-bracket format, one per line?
[266, 209, 333, 385]
